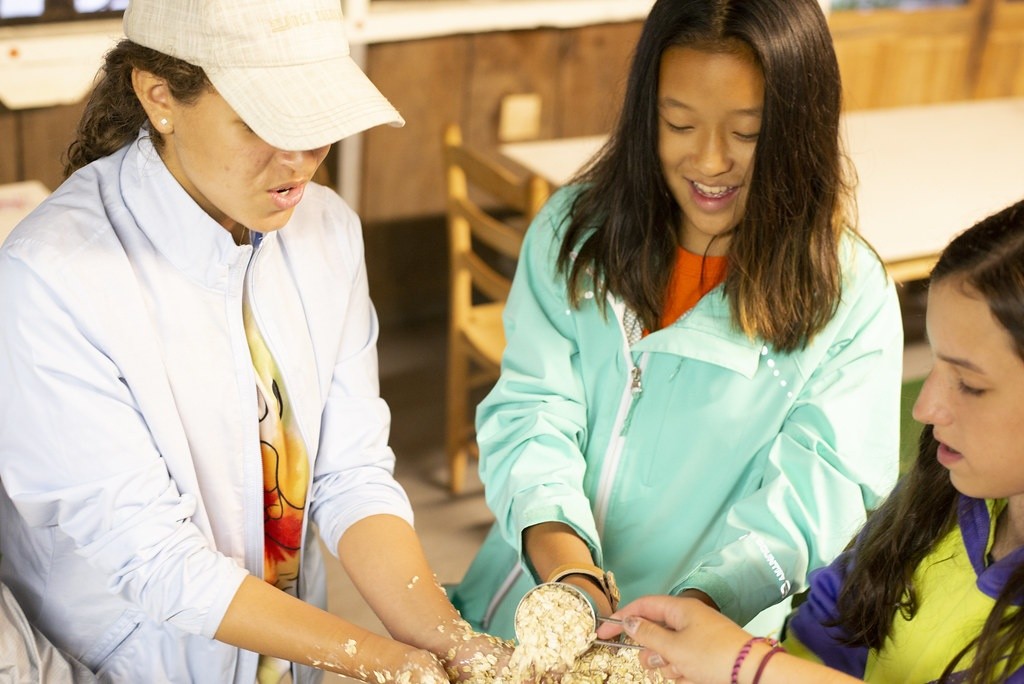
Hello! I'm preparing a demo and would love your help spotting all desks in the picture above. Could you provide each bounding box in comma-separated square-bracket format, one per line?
[496, 95, 1024, 284]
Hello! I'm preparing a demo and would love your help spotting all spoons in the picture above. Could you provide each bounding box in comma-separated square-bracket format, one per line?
[515, 582, 645, 655]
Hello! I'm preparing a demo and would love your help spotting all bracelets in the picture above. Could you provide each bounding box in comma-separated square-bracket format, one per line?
[753, 647, 787, 684]
[732, 636, 777, 684]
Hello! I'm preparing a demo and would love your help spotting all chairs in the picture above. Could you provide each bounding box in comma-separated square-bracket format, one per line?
[444, 122, 548, 499]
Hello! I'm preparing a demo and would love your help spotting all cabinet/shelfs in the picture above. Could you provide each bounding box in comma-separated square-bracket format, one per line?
[0, 0, 1024, 226]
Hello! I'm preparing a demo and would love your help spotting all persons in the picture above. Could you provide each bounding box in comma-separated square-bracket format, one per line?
[778, 200, 1023, 684]
[597, 595, 866, 684]
[0, 0, 515, 684]
[449, 0, 904, 630]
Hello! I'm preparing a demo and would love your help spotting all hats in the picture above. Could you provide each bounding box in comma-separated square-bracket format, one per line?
[122, 0, 404, 151]
